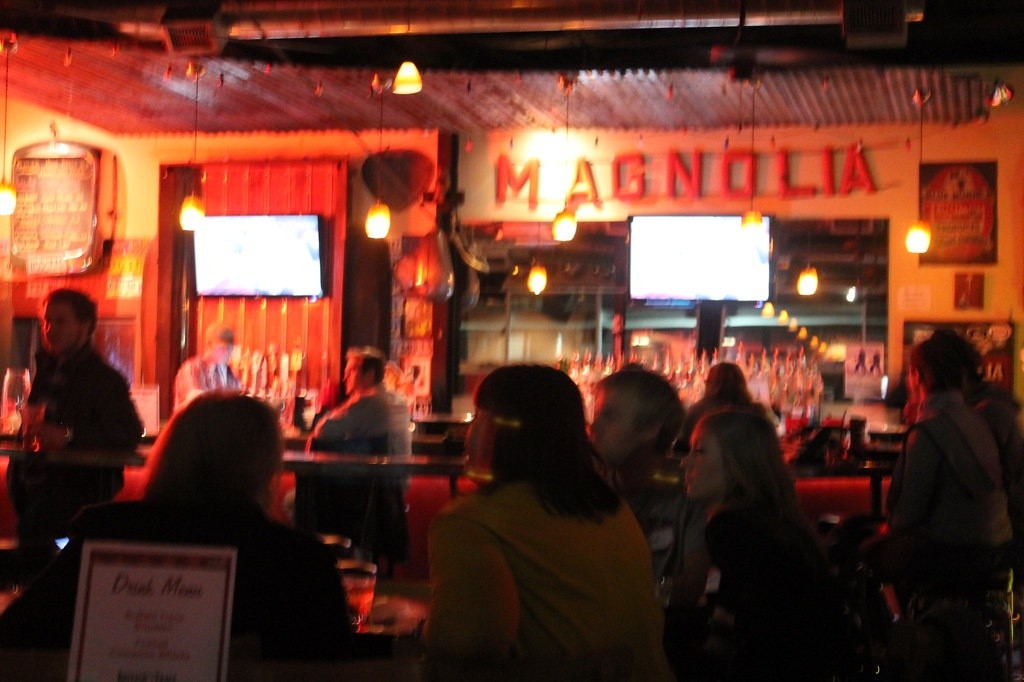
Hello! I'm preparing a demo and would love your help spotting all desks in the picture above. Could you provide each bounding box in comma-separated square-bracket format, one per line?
[0, 428, 913, 518]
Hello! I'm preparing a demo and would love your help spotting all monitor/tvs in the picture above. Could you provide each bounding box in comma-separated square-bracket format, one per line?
[194, 215, 322, 297]
[628, 215, 772, 304]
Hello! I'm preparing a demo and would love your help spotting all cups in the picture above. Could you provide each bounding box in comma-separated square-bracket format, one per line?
[337, 557, 378, 622]
[2, 367, 45, 453]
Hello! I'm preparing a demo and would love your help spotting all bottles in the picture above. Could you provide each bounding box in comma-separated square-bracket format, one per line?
[556, 339, 824, 437]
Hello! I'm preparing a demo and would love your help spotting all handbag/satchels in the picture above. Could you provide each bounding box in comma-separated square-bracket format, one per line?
[886, 415, 1014, 552]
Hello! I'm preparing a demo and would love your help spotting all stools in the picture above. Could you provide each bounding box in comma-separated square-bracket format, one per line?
[908, 568, 1013, 682]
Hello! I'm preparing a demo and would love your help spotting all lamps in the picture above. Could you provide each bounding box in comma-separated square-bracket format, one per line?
[179, 63, 205, 230]
[904, 92, 933, 254]
[392, 60, 422, 94]
[0, 37, 19, 215]
[761, 266, 825, 353]
[528, 222, 547, 295]
[366, 72, 390, 238]
[552, 75, 578, 242]
[741, 67, 764, 231]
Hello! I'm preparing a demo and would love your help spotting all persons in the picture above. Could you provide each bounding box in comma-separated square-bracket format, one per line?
[313, 345, 413, 494]
[0, 389, 363, 682]
[674, 407, 863, 682]
[856, 329, 1024, 596]
[584, 363, 695, 630]
[366, 364, 673, 681]
[674, 363, 767, 452]
[173, 322, 245, 409]
[5, 288, 145, 589]
